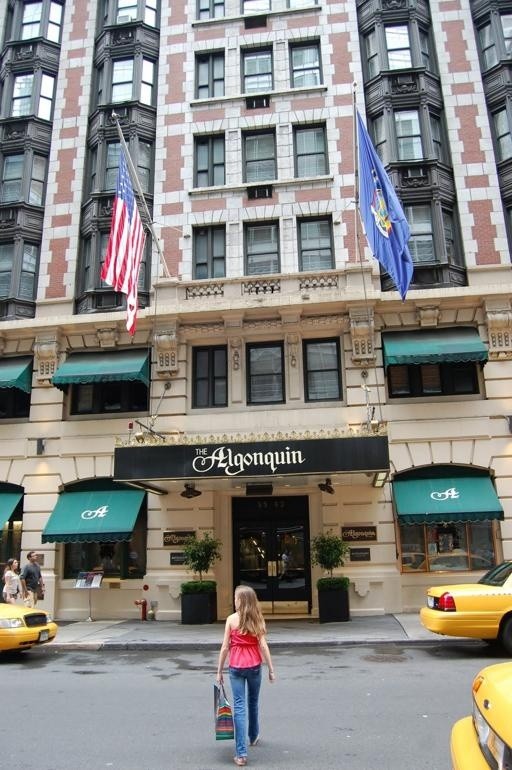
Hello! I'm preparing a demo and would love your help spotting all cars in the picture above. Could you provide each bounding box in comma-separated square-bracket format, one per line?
[400, 552, 492, 573]
[449, 663, 512, 769]
[0, 602, 57, 653]
[419, 561, 511, 654]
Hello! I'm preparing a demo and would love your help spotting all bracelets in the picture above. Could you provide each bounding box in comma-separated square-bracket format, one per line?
[269, 671, 274, 673]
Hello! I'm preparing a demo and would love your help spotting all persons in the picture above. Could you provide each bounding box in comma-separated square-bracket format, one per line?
[18, 550, 46, 607]
[216, 583, 276, 767]
[2, 558, 23, 604]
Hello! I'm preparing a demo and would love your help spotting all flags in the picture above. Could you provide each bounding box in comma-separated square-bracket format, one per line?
[100, 141, 148, 337]
[356, 113, 415, 304]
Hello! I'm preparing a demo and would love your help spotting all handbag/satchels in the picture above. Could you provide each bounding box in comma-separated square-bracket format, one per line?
[36, 584, 45, 600]
[214, 680, 235, 741]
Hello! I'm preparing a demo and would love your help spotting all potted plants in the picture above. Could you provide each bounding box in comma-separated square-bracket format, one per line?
[310, 527, 351, 625]
[179, 528, 225, 624]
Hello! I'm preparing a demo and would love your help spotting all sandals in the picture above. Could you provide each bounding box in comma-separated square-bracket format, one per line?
[233, 755, 246, 766]
